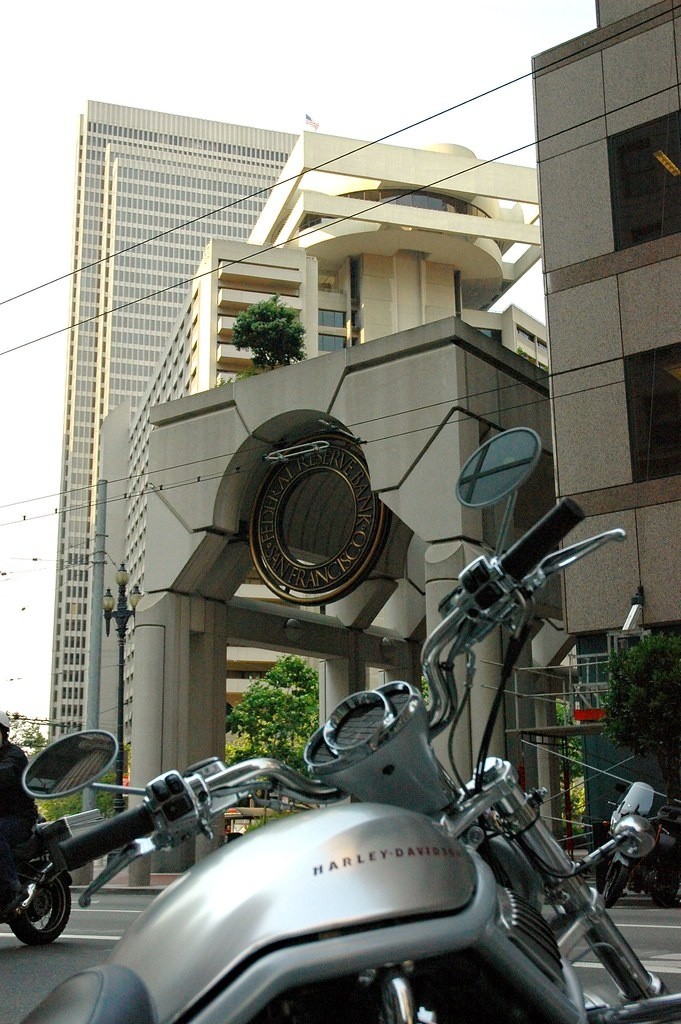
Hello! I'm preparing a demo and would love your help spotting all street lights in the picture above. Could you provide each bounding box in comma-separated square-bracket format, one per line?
[103, 562, 141, 816]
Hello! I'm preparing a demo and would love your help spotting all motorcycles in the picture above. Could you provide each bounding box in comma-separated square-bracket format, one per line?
[599, 779, 681, 910]
[0, 806, 104, 944]
[6, 422, 680, 1024]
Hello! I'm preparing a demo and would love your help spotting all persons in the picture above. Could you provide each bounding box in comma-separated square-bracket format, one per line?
[0, 725, 38, 915]
[225, 825, 231, 833]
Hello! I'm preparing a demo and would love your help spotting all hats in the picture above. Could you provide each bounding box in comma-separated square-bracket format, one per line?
[0, 722, 10, 744]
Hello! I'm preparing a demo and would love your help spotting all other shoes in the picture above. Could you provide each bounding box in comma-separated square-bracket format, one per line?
[0, 885, 30, 923]
